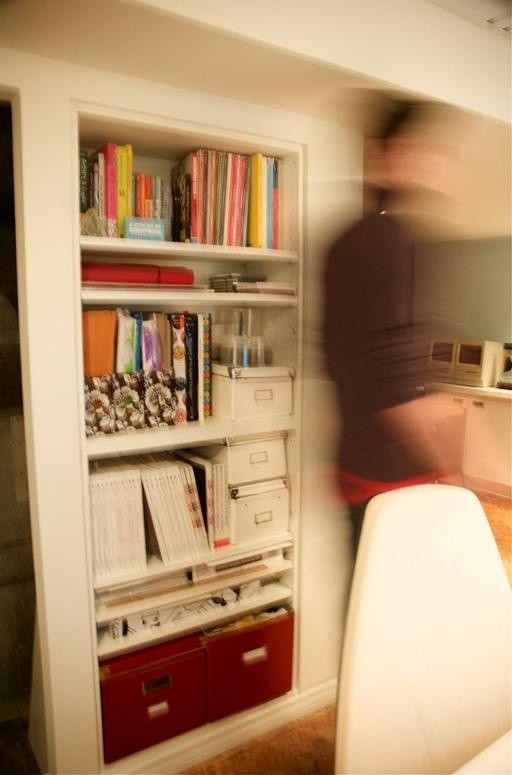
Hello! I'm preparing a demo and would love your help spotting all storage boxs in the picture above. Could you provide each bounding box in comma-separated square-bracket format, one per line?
[212, 364, 295, 423]
[97, 636, 204, 765]
[204, 607, 297, 726]
[217, 435, 288, 488]
[214, 476, 293, 548]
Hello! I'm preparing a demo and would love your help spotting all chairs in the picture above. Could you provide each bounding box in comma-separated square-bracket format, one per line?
[334, 482, 508, 774]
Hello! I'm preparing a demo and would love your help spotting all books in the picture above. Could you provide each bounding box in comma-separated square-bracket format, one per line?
[90, 455, 230, 588]
[84, 305, 214, 424]
[83, 256, 297, 293]
[80, 140, 282, 248]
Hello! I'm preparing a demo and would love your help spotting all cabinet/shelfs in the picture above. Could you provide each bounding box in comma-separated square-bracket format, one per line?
[68, 102, 308, 766]
